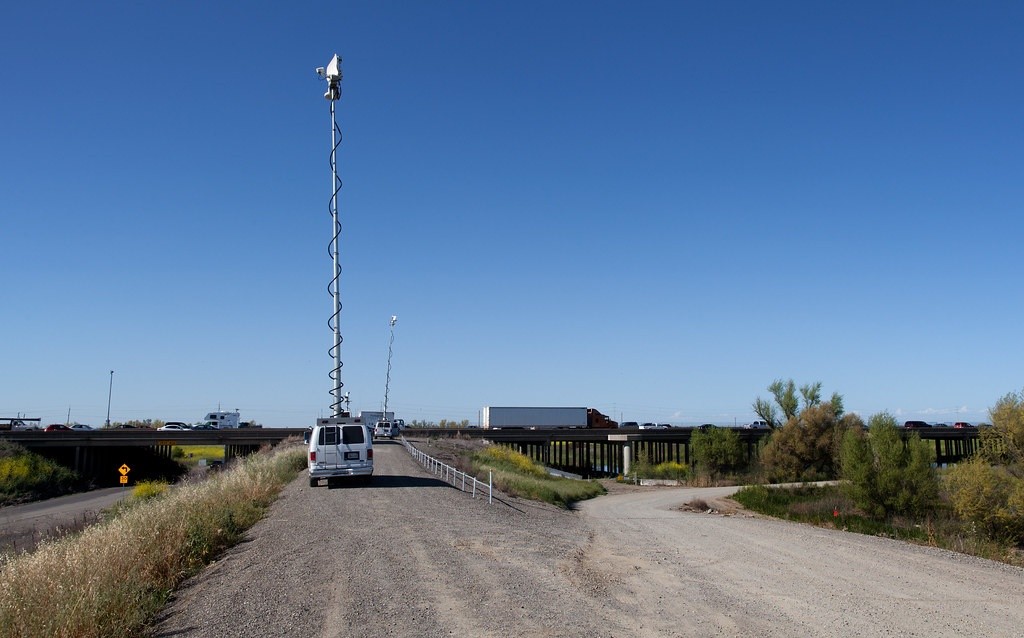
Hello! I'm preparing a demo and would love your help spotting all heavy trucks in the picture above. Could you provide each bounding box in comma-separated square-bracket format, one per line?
[483, 405, 620, 431]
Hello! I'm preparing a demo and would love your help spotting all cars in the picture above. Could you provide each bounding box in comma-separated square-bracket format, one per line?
[954, 422, 974, 428]
[698, 423, 717, 428]
[620, 421, 671, 431]
[0, 418, 220, 433]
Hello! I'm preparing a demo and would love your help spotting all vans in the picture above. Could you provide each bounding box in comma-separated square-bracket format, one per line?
[749, 421, 774, 430]
[374, 421, 393, 439]
[905, 421, 932, 428]
[304, 419, 374, 488]
